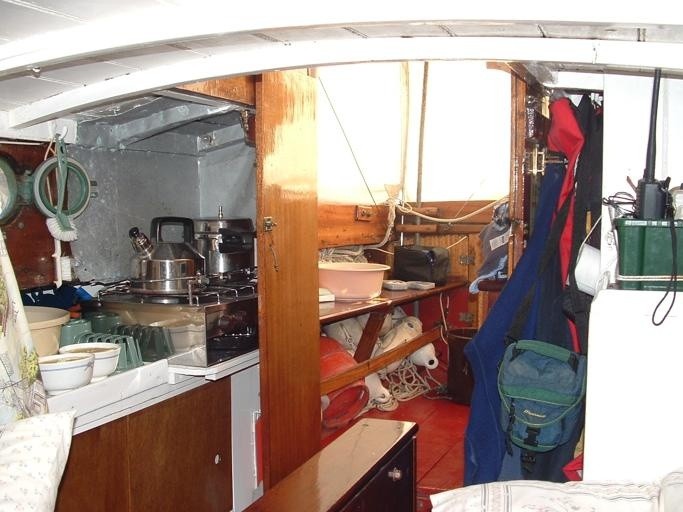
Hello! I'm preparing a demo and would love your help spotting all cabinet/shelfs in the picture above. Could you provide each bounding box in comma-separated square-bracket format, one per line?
[52, 373, 232, 512]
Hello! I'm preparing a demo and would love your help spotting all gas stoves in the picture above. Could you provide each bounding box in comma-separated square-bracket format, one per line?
[83, 260, 258, 368]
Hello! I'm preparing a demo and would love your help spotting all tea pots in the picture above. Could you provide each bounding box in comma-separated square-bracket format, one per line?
[128, 214, 206, 296]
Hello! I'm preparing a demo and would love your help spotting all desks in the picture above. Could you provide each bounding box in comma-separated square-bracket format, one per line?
[318, 276, 472, 441]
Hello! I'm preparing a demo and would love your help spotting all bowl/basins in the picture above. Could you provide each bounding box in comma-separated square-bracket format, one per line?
[57, 342, 121, 383]
[21, 304, 71, 356]
[317, 261, 392, 303]
[36, 351, 96, 399]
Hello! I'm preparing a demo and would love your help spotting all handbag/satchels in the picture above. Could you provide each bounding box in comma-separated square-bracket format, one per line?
[561, 287, 594, 321]
[526, 108, 551, 145]
[562, 244, 601, 296]
[478, 205, 511, 280]
[393, 245, 449, 285]
[497, 340, 587, 452]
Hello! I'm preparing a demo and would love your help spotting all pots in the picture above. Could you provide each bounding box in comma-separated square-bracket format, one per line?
[187, 207, 256, 281]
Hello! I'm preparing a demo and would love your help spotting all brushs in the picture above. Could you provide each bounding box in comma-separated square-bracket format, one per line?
[45, 133, 78, 242]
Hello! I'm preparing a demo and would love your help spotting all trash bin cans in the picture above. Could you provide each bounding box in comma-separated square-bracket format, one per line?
[446, 328, 479, 404]
